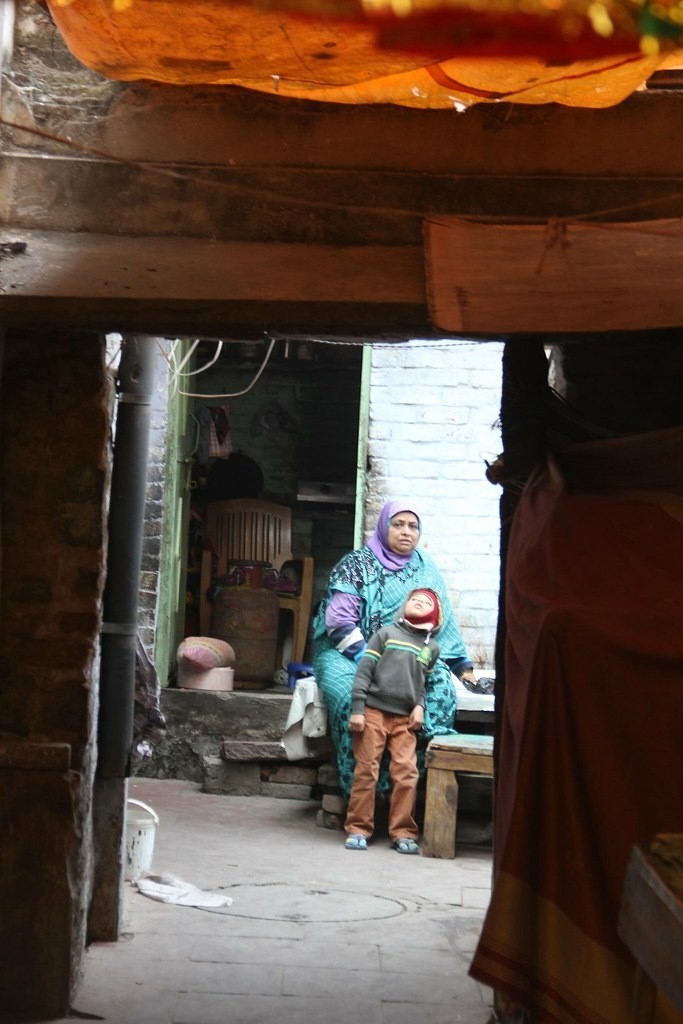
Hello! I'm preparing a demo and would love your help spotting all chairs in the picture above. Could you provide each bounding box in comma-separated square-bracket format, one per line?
[194, 497, 313, 692]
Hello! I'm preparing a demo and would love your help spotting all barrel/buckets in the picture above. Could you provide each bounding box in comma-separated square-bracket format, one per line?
[123, 798, 159, 880]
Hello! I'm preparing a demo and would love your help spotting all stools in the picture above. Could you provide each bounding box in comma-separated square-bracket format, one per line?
[285, 662, 314, 687]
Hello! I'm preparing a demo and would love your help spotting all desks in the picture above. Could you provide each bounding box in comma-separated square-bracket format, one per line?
[419, 731, 497, 859]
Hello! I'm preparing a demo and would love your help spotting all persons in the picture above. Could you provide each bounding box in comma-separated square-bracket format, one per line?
[343, 586, 444, 856]
[311, 497, 478, 816]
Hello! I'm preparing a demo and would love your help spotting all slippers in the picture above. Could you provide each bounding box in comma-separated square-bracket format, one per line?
[392, 837, 419, 855]
[343, 833, 370, 851]
